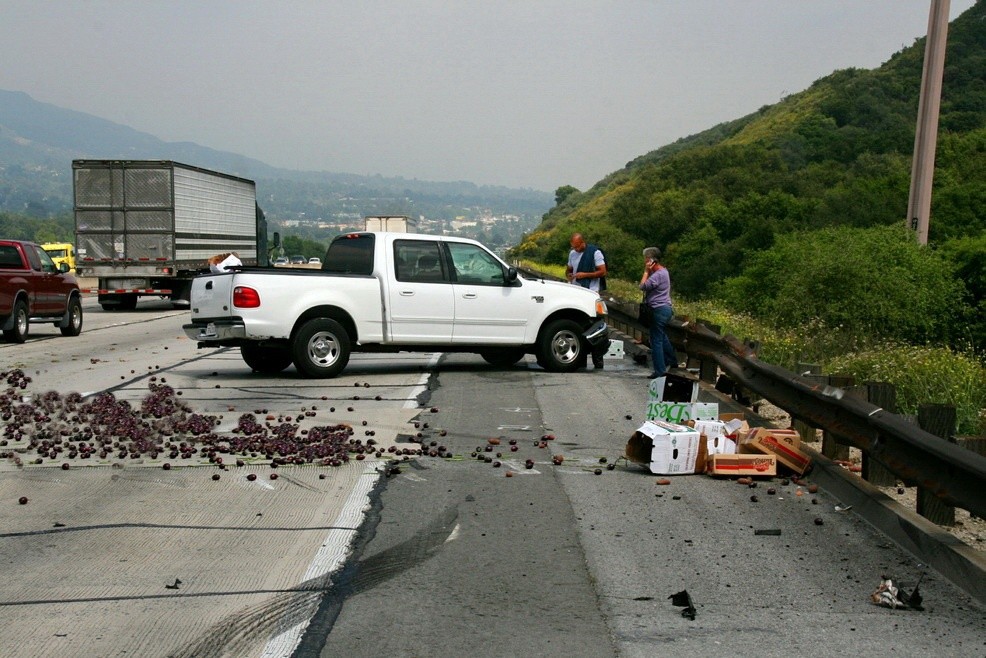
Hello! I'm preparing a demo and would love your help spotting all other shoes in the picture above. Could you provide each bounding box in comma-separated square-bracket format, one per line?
[647, 373, 657, 379]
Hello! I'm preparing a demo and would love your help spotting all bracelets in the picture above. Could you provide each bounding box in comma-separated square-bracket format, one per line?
[644, 271, 649, 275]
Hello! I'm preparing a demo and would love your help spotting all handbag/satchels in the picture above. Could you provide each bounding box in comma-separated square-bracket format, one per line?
[638, 303, 649, 328]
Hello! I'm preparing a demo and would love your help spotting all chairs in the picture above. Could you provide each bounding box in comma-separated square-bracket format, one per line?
[413, 254, 441, 281]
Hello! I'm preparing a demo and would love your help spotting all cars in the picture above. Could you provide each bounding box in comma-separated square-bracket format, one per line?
[290, 255, 307, 265]
[308, 257, 321, 264]
[274, 258, 287, 267]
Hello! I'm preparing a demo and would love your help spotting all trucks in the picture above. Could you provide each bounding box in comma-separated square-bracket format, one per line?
[71, 158, 279, 312]
[365, 215, 418, 265]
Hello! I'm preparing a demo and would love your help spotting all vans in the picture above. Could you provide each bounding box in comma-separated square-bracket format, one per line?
[37, 241, 76, 273]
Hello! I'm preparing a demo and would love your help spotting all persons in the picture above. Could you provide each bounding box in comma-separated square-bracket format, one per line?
[638, 247, 678, 379]
[565, 232, 607, 368]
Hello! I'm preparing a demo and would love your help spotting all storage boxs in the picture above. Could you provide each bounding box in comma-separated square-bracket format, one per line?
[624, 367, 811, 477]
[603, 339, 624, 359]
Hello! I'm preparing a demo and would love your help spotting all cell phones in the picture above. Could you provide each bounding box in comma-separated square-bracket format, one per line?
[651, 260, 658, 265]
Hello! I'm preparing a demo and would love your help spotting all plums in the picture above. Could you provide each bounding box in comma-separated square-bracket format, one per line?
[898, 487, 905, 494]
[0, 369, 826, 527]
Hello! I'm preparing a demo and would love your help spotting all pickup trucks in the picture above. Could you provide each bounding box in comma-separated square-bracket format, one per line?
[0, 239, 84, 345]
[181, 231, 611, 380]
